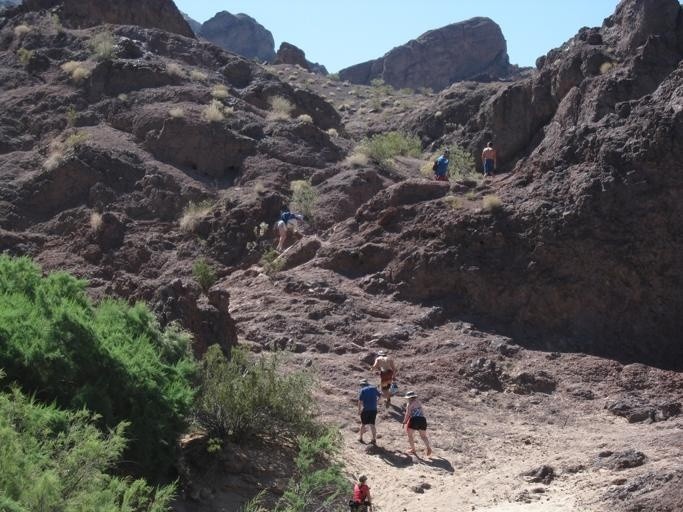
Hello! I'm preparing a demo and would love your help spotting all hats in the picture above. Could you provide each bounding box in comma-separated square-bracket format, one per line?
[378, 351, 386, 356]
[405, 391, 418, 398]
[360, 379, 368, 385]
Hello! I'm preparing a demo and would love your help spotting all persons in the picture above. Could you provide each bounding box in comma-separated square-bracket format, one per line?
[357, 379, 380, 442]
[482, 141, 497, 177]
[434, 150, 450, 180]
[401, 391, 432, 456]
[276, 212, 303, 252]
[351, 474, 372, 512]
[373, 351, 396, 408]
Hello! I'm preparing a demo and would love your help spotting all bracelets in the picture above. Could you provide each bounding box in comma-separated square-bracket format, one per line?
[402, 422, 405, 425]
[358, 409, 360, 410]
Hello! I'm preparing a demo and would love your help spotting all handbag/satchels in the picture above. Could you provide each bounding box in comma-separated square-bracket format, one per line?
[389, 383, 399, 395]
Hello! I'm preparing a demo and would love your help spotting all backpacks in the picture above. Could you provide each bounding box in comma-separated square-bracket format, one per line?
[281, 211, 296, 224]
